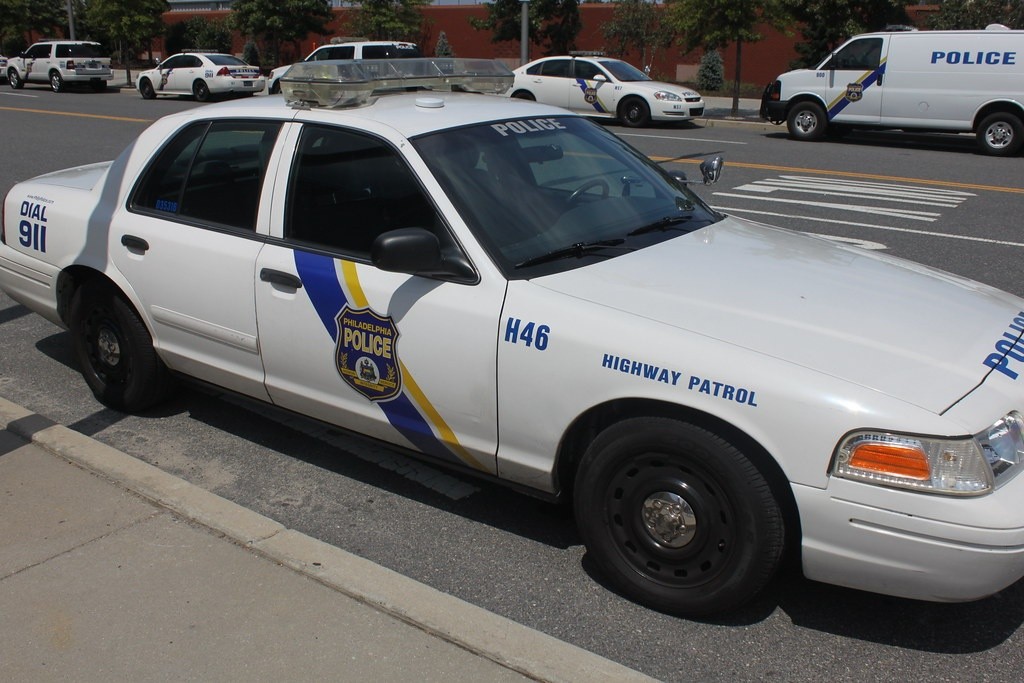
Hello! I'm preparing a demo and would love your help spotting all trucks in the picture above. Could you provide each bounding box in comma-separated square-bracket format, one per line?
[760, 22, 1023, 157]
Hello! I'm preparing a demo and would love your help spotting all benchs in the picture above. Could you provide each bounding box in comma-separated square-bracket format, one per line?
[167, 155, 402, 230]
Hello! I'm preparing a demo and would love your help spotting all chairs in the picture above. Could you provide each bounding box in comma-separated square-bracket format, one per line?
[315, 144, 414, 251]
[435, 134, 530, 235]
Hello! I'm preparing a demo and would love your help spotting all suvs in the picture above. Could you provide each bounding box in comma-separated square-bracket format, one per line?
[4, 38, 115, 93]
[267, 36, 422, 96]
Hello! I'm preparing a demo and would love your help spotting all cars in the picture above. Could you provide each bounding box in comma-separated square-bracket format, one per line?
[135, 50, 266, 104]
[483, 50, 706, 129]
[1, 56, 1024, 623]
